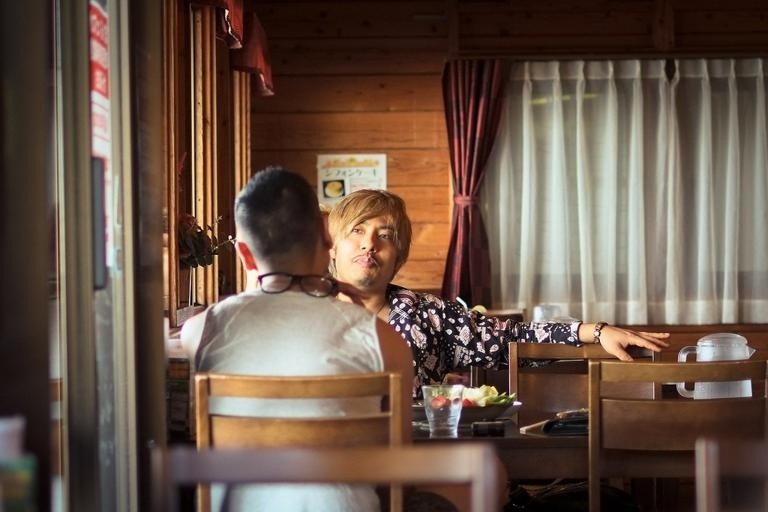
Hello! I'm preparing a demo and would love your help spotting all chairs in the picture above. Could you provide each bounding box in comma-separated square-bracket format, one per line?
[152, 441, 507, 512]
[588, 359, 768, 512]
[510, 341, 663, 407]
[195, 373, 410, 512]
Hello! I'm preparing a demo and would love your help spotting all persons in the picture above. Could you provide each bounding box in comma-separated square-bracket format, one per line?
[323, 190, 671, 396]
[180, 163, 413, 512]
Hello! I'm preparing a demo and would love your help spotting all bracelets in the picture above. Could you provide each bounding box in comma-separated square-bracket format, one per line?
[591, 320, 608, 344]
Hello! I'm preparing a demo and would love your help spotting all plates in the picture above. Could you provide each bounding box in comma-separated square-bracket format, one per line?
[412, 398, 523, 420]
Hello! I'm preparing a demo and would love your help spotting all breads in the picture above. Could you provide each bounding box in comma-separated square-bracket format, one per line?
[327, 181, 342, 195]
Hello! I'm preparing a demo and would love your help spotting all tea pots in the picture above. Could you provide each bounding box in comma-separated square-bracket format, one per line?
[676, 332, 757, 400]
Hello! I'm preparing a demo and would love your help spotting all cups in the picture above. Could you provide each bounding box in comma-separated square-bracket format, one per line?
[422, 384, 464, 435]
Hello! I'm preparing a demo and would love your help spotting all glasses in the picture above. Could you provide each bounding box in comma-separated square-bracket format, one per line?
[257, 272, 335, 298]
